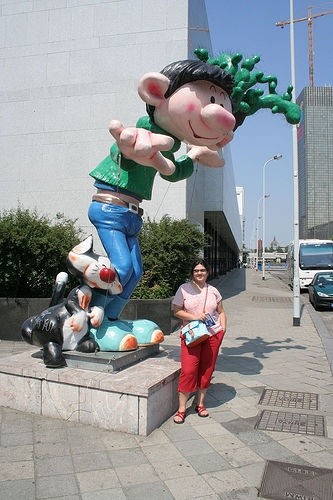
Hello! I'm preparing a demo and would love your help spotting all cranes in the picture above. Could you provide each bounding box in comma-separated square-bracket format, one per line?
[275, 7, 333, 88]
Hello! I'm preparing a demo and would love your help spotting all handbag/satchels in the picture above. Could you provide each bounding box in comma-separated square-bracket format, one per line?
[181, 321, 211, 348]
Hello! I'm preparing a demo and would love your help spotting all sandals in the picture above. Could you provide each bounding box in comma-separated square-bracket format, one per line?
[195, 406, 209, 417]
[173, 412, 185, 423]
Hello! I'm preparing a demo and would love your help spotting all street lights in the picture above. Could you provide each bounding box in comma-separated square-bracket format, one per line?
[256, 194, 271, 271]
[262, 154, 283, 280]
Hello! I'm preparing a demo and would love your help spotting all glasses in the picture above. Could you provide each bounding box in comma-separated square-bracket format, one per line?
[193, 269, 207, 272]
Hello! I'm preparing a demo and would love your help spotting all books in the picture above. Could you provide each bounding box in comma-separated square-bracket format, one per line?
[203, 313, 216, 328]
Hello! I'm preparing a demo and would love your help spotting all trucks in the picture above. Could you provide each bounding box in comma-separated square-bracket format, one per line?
[287, 239, 333, 292]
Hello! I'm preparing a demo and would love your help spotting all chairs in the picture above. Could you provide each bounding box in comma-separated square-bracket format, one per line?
[319, 277, 326, 284]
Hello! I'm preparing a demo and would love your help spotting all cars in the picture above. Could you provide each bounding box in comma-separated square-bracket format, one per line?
[305, 271, 333, 311]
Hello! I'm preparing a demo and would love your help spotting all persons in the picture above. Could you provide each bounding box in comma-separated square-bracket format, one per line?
[171, 259, 226, 424]
[88, 61, 237, 352]
